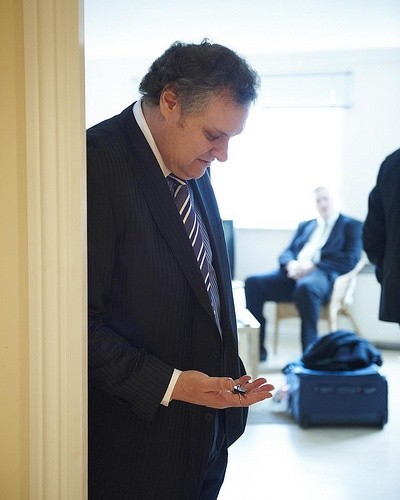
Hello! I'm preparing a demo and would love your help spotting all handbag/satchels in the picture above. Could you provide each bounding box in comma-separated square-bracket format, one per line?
[281, 329, 383, 376]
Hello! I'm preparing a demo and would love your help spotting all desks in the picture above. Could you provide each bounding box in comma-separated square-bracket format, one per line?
[236, 307, 261, 379]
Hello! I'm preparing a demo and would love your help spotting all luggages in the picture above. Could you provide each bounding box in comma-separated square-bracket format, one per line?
[286, 363, 389, 430]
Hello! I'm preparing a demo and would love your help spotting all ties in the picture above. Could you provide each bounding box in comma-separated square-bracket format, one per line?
[167, 173, 222, 341]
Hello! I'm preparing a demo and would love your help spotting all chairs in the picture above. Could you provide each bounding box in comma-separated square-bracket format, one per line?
[273, 250, 367, 357]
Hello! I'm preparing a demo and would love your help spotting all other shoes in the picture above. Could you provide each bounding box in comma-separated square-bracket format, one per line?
[258, 347, 267, 361]
